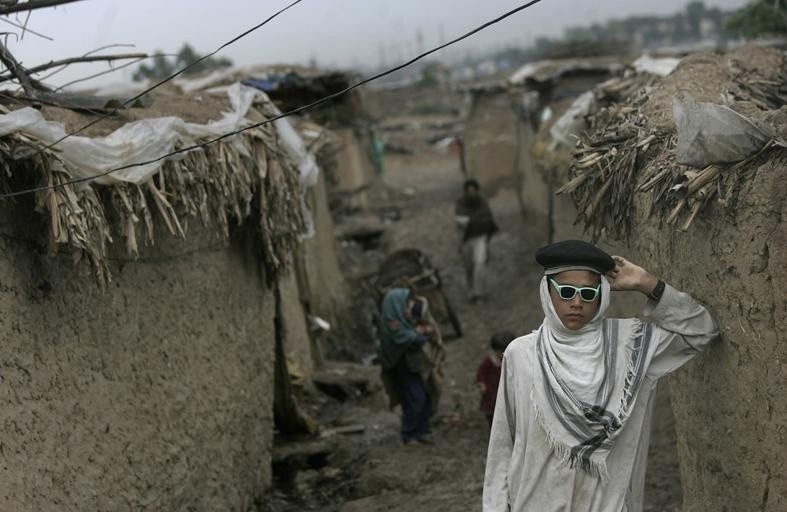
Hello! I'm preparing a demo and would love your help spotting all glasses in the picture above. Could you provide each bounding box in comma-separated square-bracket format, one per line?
[549, 278, 601, 303]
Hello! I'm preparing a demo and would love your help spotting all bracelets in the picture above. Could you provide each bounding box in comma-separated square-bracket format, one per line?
[647, 280, 665, 302]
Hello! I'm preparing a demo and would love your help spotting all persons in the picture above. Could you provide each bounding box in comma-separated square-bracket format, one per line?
[482, 240, 717, 512]
[455, 179, 498, 300]
[478, 332, 517, 431]
[378, 288, 448, 448]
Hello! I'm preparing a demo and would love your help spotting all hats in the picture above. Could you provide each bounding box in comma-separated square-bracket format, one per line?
[534, 239, 616, 276]
